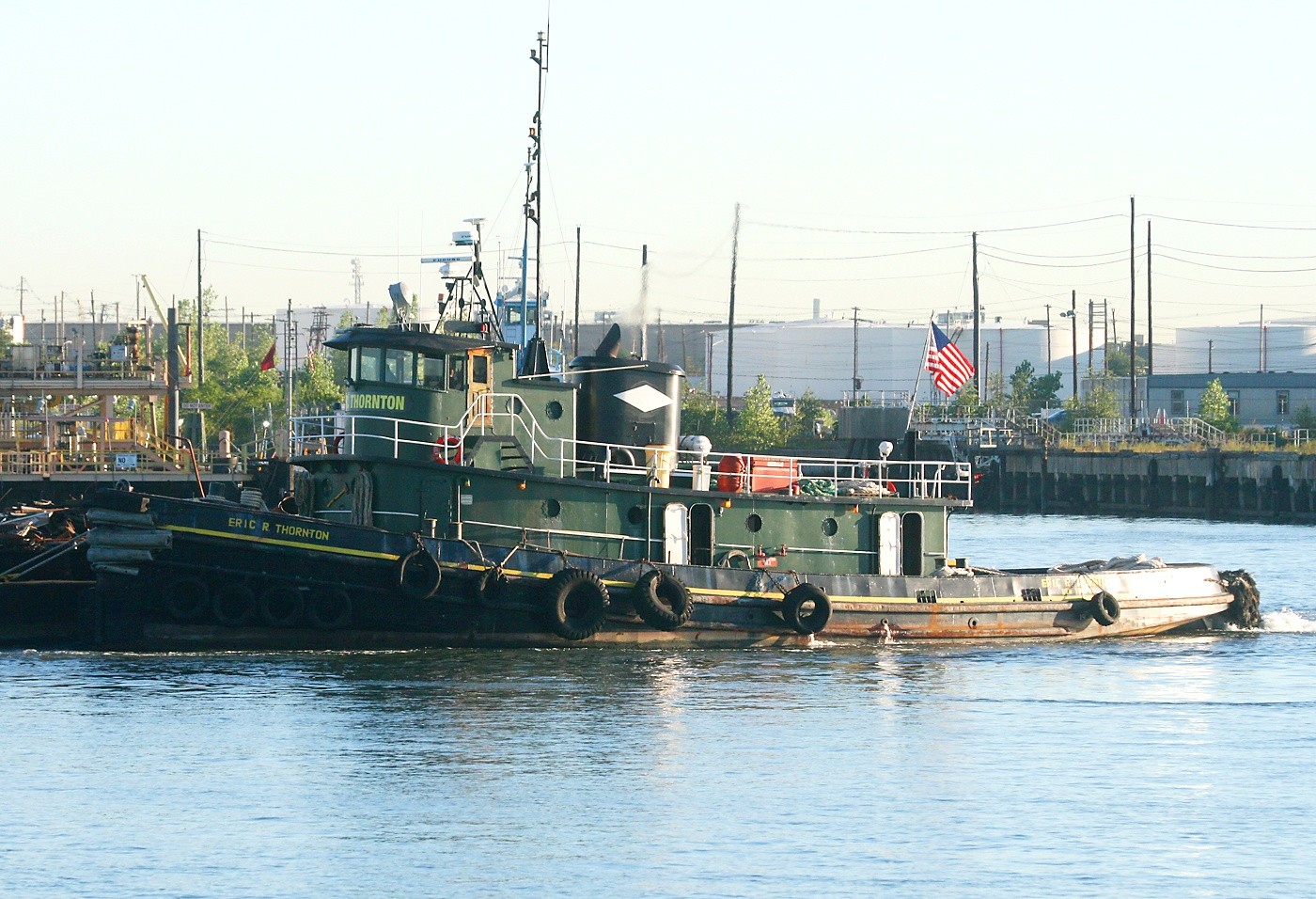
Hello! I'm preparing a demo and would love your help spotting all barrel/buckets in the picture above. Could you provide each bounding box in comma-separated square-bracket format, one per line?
[692, 464, 712, 491]
[718, 453, 745, 493]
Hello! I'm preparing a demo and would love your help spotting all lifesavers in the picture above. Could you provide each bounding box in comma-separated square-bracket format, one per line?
[332, 431, 345, 454]
[470, 567, 516, 613]
[1144, 458, 1158, 509]
[393, 547, 443, 599]
[541, 567, 613, 641]
[781, 584, 833, 635]
[1092, 591, 1121, 627]
[634, 570, 695, 632]
[432, 434, 464, 466]
[1269, 464, 1284, 516]
[163, 575, 353, 629]
[609, 447, 636, 467]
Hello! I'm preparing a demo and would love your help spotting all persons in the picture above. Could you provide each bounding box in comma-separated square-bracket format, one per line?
[434, 368, 457, 390]
[385, 364, 395, 383]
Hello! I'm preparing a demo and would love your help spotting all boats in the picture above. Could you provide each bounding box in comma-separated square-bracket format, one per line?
[0, 0, 1266, 651]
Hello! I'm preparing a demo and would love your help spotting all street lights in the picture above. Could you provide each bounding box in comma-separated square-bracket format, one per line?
[1060, 310, 1079, 412]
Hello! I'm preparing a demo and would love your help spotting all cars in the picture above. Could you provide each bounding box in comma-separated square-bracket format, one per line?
[1035, 408, 1093, 432]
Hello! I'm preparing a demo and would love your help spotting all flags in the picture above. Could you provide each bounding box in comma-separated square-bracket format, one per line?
[306, 341, 315, 377]
[924, 321, 975, 397]
[260, 340, 276, 371]
